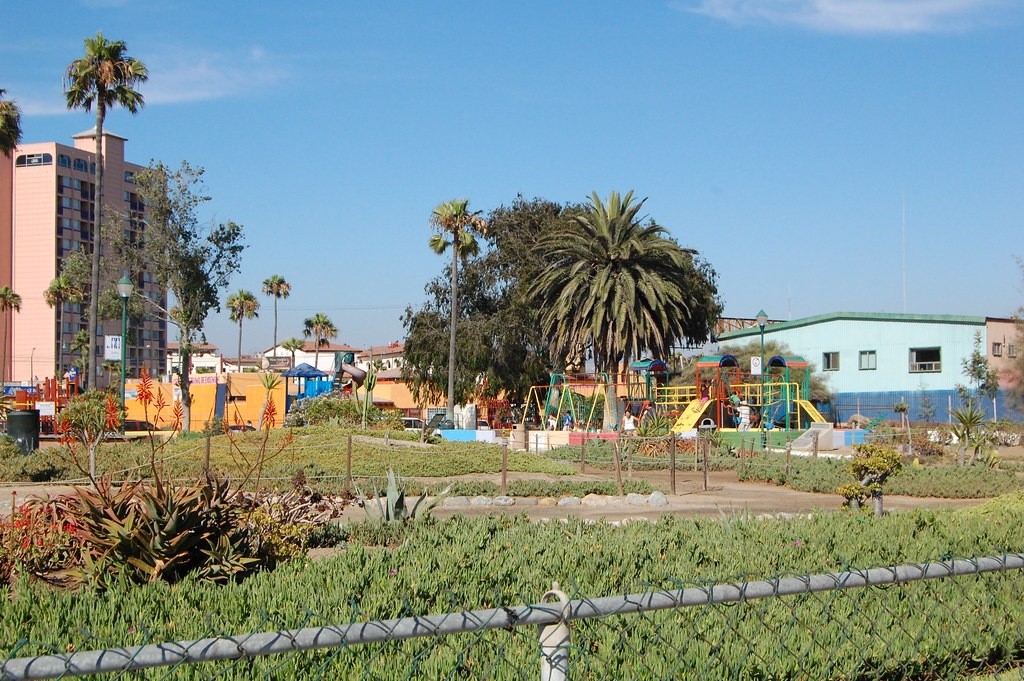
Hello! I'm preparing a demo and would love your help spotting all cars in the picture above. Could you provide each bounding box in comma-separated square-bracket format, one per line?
[476, 420, 492, 432]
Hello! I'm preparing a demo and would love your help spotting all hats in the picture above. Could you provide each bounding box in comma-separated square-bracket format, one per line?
[731, 390, 736, 394]
[740, 401, 747, 405]
[566, 410, 571, 414]
[643, 400, 652, 406]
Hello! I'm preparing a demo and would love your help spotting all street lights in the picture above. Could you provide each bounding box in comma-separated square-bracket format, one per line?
[30, 347, 38, 395]
[116, 273, 134, 435]
[754, 309, 769, 450]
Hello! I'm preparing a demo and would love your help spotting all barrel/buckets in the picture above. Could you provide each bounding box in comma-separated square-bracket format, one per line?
[7, 408, 40, 450]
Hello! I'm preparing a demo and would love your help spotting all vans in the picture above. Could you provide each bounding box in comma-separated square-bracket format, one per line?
[372, 417, 443, 440]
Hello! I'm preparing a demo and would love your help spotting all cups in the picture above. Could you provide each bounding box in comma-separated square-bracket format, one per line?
[597, 429, 601, 433]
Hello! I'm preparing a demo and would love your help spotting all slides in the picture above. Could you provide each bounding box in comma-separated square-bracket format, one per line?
[792, 399, 828, 424]
[214, 383, 227, 419]
[341, 363, 367, 394]
[666, 398, 718, 435]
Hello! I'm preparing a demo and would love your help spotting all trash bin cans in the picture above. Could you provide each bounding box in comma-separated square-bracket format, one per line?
[698, 418, 717, 437]
[6, 409, 40, 456]
[510, 424, 526, 451]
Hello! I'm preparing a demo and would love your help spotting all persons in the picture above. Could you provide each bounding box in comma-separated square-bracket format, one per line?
[563, 410, 574, 431]
[621, 410, 639, 434]
[643, 400, 654, 427]
[727, 401, 750, 432]
[771, 370, 780, 390]
[726, 391, 741, 407]
[546, 414, 556, 431]
[693, 379, 713, 413]
